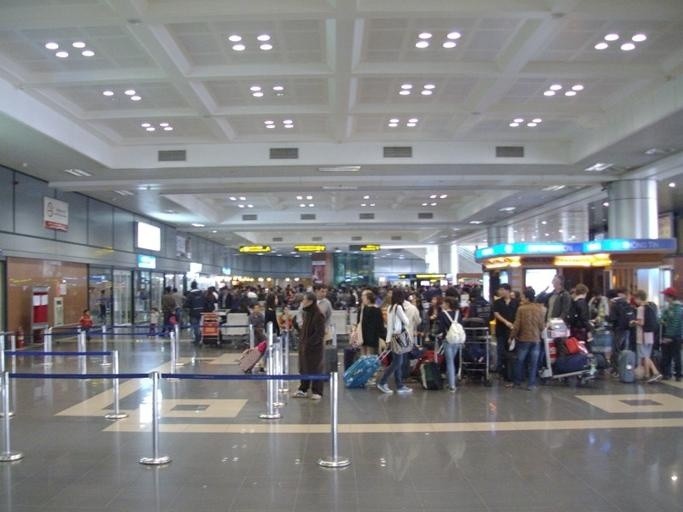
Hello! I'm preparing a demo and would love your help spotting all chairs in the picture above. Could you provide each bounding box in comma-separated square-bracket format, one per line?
[221, 301, 358, 349]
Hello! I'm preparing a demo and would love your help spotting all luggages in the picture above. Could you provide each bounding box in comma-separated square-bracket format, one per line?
[324, 343, 338, 373]
[503, 333, 526, 383]
[414, 332, 446, 389]
[343, 346, 392, 388]
[619, 330, 636, 382]
[652, 323, 669, 380]
[239, 341, 266, 373]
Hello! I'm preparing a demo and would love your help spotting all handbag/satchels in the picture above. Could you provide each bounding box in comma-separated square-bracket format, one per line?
[390, 329, 419, 360]
[348, 323, 364, 349]
[431, 319, 440, 333]
[555, 337, 590, 372]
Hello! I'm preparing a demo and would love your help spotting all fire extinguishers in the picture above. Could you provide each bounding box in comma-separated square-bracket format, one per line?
[17, 326, 25, 348]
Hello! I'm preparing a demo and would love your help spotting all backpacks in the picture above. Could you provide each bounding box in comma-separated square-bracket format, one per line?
[615, 299, 635, 330]
[472, 298, 491, 318]
[441, 309, 467, 344]
[640, 302, 657, 331]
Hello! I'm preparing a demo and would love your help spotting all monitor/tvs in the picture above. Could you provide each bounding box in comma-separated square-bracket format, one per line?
[190, 262, 203, 273]
[137, 254, 157, 268]
[221, 267, 232, 275]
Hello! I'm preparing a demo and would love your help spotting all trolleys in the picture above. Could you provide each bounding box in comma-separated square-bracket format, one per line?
[162, 309, 190, 336]
[199, 312, 221, 347]
[457, 317, 493, 386]
[537, 328, 598, 387]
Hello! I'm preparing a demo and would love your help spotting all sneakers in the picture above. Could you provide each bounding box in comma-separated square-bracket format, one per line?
[291, 389, 322, 400]
[644, 374, 681, 383]
[396, 385, 413, 393]
[505, 383, 537, 391]
[376, 383, 393, 393]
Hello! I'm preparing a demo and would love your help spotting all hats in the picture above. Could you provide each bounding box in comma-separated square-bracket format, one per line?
[659, 288, 679, 296]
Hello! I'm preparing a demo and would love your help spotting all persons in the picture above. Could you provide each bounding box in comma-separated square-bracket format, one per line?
[95, 290, 108, 318]
[149, 281, 683, 401]
[80, 309, 93, 342]
